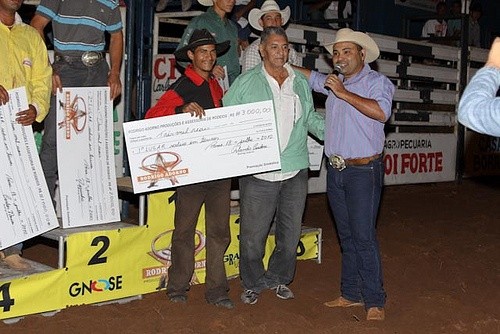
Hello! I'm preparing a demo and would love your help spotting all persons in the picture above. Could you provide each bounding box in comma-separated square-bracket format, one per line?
[146, 29, 234, 310]
[244, 12, 303, 76]
[456, 36, 500, 137]
[288, 28, 394, 321]
[0, 0, 53, 273]
[221, 26, 325, 306]
[30, 0, 124, 219]
[174, 0, 243, 89]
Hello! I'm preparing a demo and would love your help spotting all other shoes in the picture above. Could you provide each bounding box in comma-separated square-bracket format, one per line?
[214, 299, 235, 310]
[323, 295, 366, 308]
[3, 254, 32, 272]
[167, 291, 188, 303]
[364, 305, 385, 322]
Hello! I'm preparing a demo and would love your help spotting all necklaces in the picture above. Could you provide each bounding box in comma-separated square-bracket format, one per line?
[7, 22, 15, 28]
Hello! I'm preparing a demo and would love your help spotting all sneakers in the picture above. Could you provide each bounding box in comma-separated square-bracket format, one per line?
[240, 287, 261, 304]
[270, 282, 295, 300]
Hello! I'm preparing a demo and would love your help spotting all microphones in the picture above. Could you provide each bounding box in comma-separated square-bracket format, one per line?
[324, 66, 342, 90]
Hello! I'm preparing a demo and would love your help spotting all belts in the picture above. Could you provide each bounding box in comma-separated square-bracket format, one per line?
[328, 153, 382, 171]
[53, 52, 105, 67]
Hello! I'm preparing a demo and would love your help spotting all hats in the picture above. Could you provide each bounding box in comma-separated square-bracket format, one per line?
[197, 0, 251, 8]
[322, 28, 380, 64]
[248, 0, 291, 31]
[174, 28, 231, 62]
